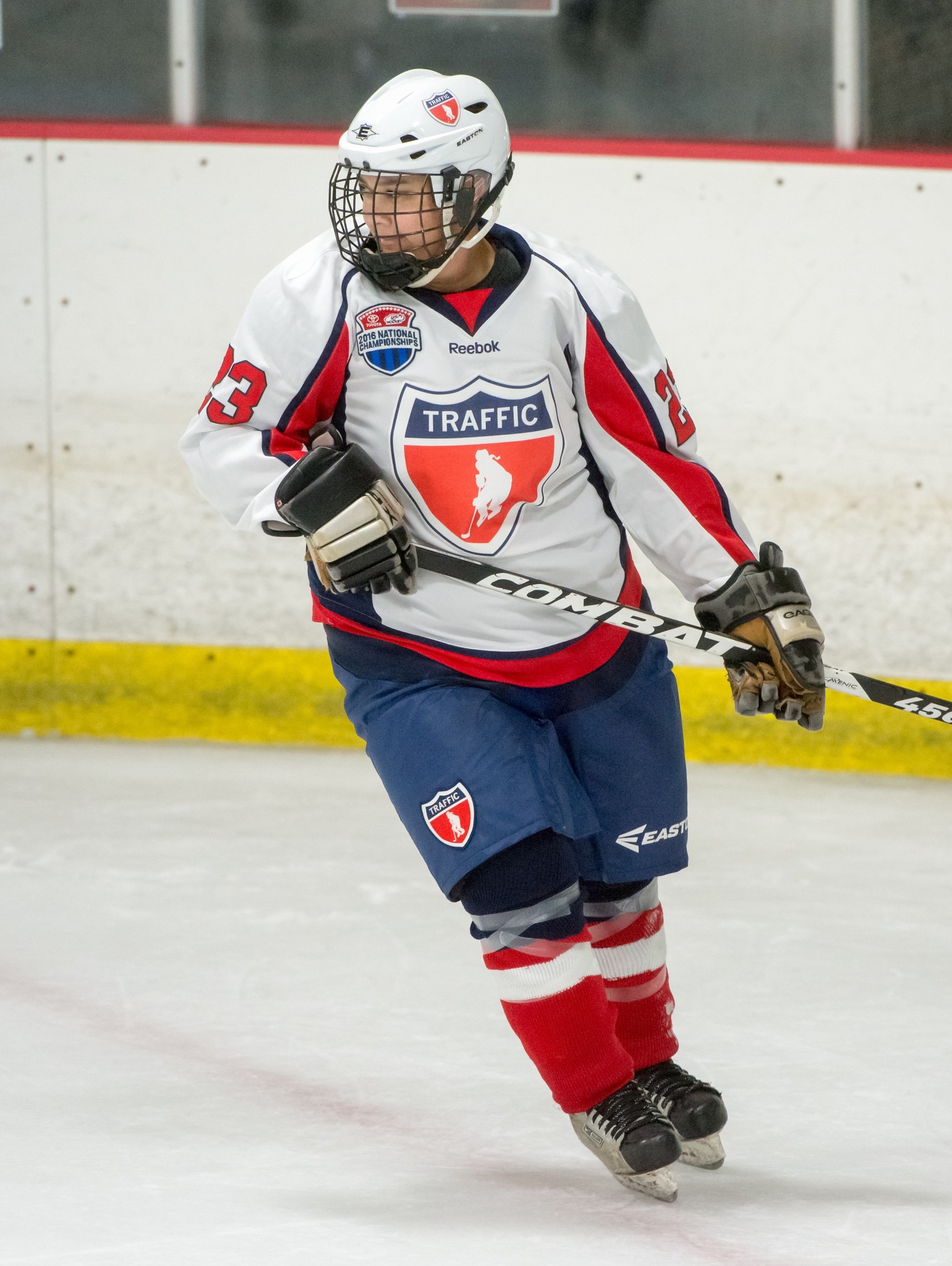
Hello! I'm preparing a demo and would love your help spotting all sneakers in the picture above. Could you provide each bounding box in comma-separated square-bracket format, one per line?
[570, 1079, 683, 1203]
[632, 1058, 728, 1170]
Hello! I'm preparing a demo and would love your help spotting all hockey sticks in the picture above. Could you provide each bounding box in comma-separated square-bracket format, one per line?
[408, 541, 952, 724]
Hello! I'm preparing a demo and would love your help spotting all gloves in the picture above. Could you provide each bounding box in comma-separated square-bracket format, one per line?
[694, 542, 825, 731]
[260, 424, 418, 596]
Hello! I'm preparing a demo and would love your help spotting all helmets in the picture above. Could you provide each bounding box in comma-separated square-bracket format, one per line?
[328, 69, 515, 294]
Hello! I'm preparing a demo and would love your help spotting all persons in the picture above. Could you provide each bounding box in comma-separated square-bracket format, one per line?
[181, 67, 830, 1208]
